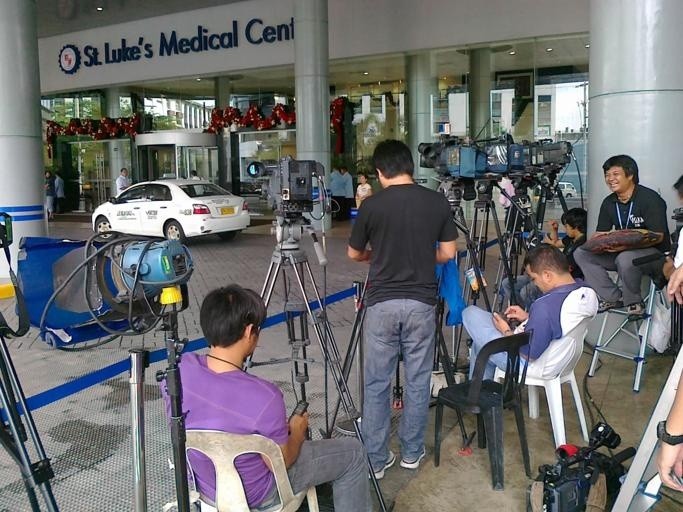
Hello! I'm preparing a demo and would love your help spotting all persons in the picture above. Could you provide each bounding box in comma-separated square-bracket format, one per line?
[329, 166, 373, 222]
[461, 243, 591, 381]
[189, 169, 206, 195]
[667, 262, 683, 305]
[158, 285, 374, 511]
[347, 139, 458, 481]
[656, 369, 683, 492]
[116, 167, 130, 197]
[45, 170, 65, 222]
[502, 207, 587, 309]
[572, 155, 670, 321]
[662, 174, 683, 282]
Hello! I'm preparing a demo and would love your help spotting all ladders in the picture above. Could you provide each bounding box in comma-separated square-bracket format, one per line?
[587, 271, 669, 393]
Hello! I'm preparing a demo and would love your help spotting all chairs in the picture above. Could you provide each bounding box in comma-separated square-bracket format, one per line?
[493, 314, 594, 450]
[435, 327, 533, 490]
[181, 427, 318, 512]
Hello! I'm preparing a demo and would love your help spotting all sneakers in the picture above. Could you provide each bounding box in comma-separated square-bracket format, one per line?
[368, 450, 395, 479]
[399, 443, 425, 469]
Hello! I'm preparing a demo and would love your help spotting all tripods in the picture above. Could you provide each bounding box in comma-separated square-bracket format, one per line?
[241, 242, 424, 512]
[458, 202, 532, 315]
[327, 267, 477, 453]
[440, 205, 499, 369]
[492, 206, 540, 313]
[538, 183, 575, 235]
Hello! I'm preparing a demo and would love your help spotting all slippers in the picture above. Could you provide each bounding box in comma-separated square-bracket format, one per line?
[597, 291, 623, 313]
[626, 298, 645, 315]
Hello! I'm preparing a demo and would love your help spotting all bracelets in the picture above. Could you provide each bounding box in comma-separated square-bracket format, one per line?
[503, 330, 513, 335]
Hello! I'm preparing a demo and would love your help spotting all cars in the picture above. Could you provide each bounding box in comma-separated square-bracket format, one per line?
[91, 177, 252, 242]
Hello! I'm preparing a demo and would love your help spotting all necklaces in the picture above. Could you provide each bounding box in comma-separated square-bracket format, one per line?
[205, 353, 243, 371]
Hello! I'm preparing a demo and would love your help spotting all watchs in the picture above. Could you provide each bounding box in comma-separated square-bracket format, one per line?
[657, 421, 683, 446]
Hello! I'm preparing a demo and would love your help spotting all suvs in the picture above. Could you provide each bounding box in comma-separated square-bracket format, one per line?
[551, 181, 578, 198]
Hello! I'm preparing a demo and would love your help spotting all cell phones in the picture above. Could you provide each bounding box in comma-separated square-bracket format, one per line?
[495, 311, 511, 321]
[286, 397, 310, 430]
